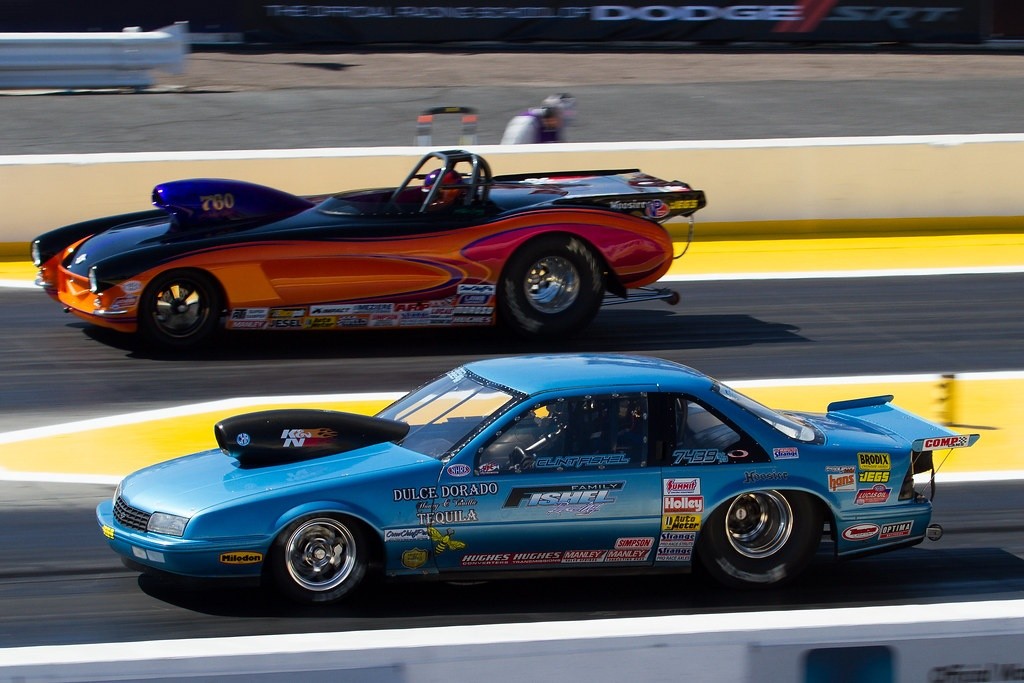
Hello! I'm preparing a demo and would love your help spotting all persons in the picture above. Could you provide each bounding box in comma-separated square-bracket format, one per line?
[500, 91, 583, 146]
[415, 168, 464, 215]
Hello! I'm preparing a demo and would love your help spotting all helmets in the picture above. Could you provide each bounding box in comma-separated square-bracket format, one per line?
[420, 168, 464, 212]
[542, 93, 576, 131]
[536, 397, 602, 454]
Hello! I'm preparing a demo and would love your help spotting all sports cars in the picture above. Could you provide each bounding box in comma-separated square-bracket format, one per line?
[25, 142, 706, 352]
[92, 352, 983, 603]
